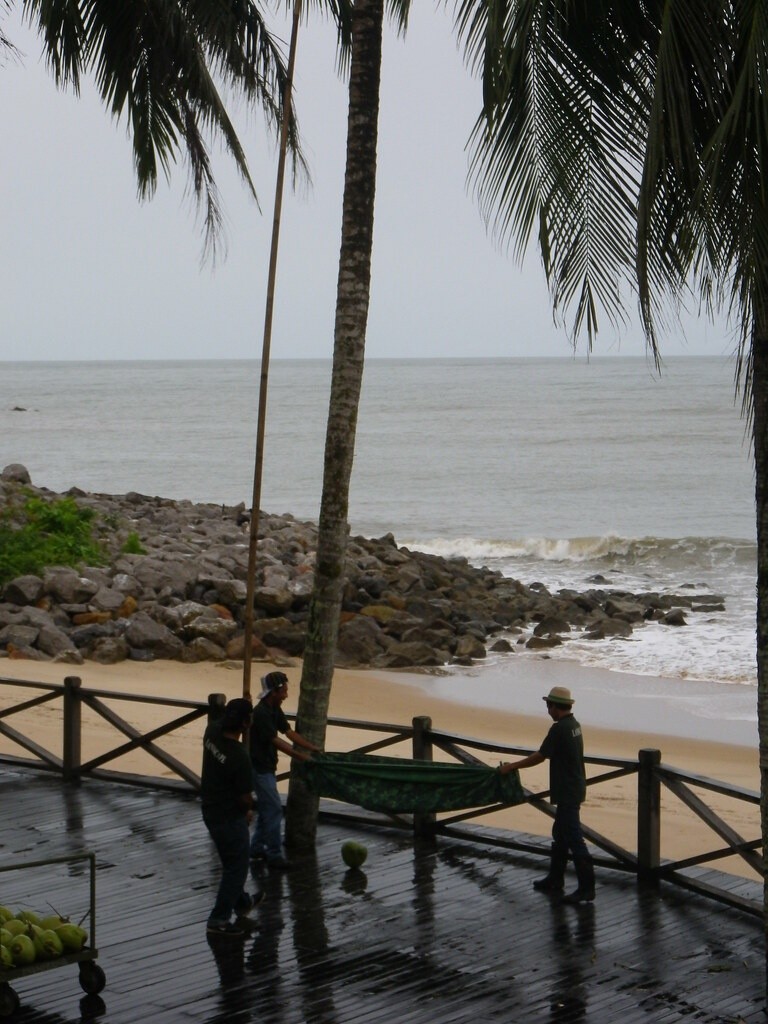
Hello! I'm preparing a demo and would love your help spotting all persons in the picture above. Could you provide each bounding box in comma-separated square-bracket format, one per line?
[243, 672, 323, 871]
[199, 698, 268, 934]
[499, 686, 598, 903]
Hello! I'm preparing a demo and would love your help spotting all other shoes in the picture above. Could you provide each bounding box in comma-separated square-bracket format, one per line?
[560, 888, 596, 904]
[533, 876, 564, 889]
[207, 922, 244, 936]
[237, 890, 265, 916]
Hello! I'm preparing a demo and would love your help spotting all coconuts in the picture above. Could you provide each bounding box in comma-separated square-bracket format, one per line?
[0, 904, 87, 971]
[341, 841, 369, 868]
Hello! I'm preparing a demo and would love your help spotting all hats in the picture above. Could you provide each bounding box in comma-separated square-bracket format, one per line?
[543, 687, 573, 705]
[257, 671, 288, 698]
